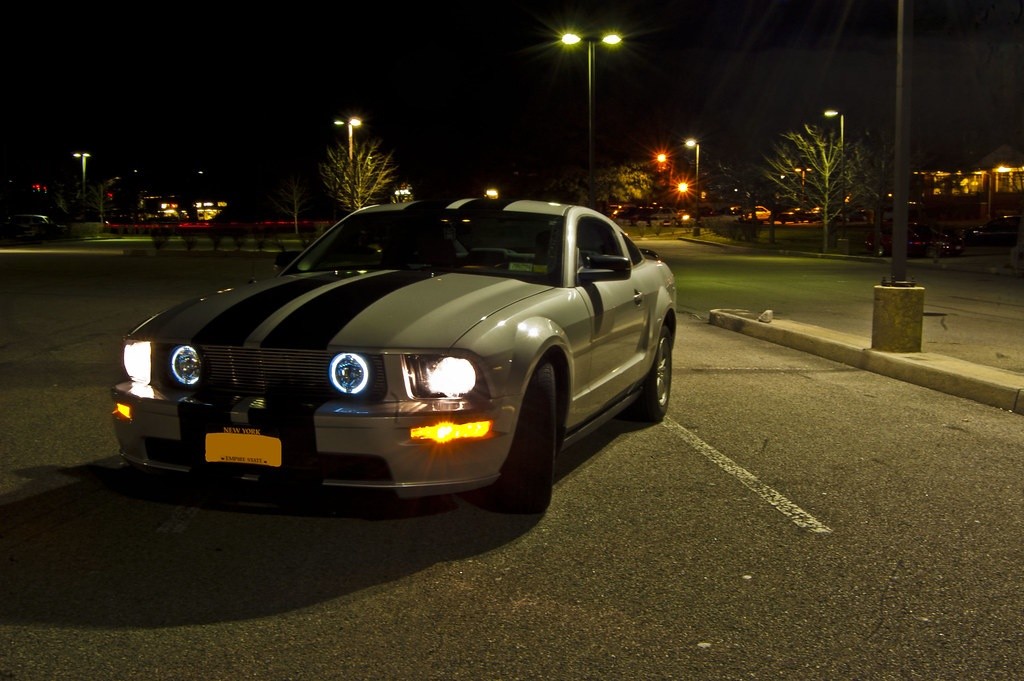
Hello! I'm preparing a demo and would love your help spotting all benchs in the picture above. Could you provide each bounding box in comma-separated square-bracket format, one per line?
[469, 247, 598, 267]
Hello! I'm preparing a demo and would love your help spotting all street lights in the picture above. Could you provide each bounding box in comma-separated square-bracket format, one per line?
[73, 151, 91, 222]
[561, 30, 624, 208]
[794, 161, 812, 223]
[682, 136, 701, 236]
[333, 114, 363, 164]
[823, 109, 848, 251]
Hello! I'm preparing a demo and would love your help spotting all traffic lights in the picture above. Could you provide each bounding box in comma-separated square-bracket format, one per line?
[36, 184, 41, 192]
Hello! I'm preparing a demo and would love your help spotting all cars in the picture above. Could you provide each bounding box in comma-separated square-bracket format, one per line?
[13, 215, 60, 241]
[112, 198, 677, 514]
[611, 204, 638, 218]
[737, 205, 771, 223]
[648, 207, 679, 220]
[777, 207, 820, 223]
[865, 221, 951, 258]
[960, 215, 1021, 247]
